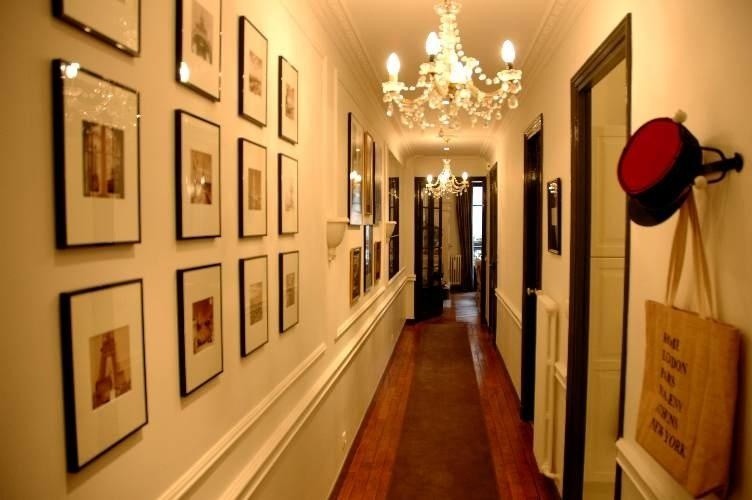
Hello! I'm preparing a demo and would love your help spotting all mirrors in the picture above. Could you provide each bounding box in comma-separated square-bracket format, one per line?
[388, 148, 400, 279]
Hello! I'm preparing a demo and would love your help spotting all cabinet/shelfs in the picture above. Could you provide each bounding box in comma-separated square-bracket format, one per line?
[583, 124, 626, 482]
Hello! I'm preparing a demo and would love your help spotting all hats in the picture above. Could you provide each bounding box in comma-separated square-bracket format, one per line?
[617, 111, 702, 226]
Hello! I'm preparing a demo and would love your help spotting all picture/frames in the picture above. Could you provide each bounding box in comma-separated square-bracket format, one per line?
[59, 279, 148, 473]
[51, 58, 141, 249]
[51, 0, 142, 56]
[545, 178, 564, 254]
[347, 113, 382, 306]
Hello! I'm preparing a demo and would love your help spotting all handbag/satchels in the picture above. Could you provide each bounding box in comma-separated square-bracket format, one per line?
[636, 300, 740, 499]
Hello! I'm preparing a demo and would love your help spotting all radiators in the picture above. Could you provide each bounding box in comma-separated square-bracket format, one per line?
[533, 291, 566, 481]
[447, 253, 462, 284]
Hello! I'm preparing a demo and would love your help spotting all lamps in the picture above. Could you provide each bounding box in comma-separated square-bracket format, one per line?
[382, 1, 523, 150]
[424, 160, 468, 200]
[386, 221, 397, 243]
[326, 221, 349, 264]
